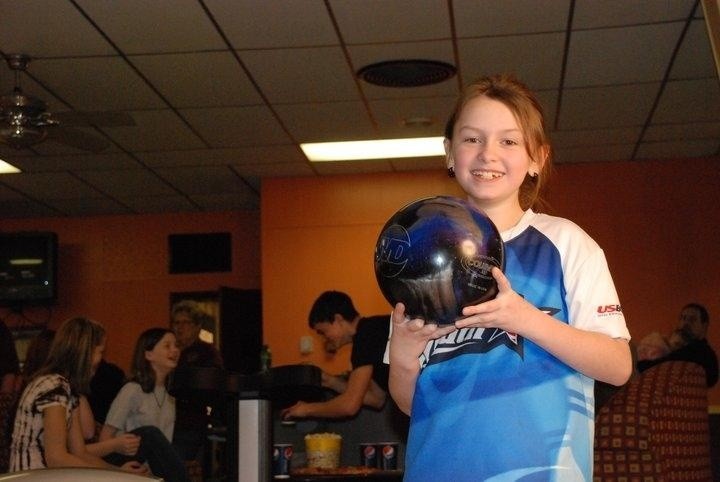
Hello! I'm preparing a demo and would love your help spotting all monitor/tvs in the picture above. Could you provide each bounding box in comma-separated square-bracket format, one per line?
[170, 233, 233, 273]
[0, 230, 59, 305]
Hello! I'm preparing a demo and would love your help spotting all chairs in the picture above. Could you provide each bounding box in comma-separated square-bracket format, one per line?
[594, 359, 716, 482]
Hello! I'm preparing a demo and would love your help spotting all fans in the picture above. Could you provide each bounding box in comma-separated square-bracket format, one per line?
[0, 53, 138, 153]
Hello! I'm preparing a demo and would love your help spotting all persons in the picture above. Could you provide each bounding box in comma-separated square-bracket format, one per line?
[389, 78, 633, 482]
[279, 290, 410, 446]
[634, 328, 692, 362]
[1, 300, 229, 481]
[636, 303, 720, 388]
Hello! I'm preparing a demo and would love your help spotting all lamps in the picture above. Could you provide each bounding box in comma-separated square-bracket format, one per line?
[0, 114, 47, 150]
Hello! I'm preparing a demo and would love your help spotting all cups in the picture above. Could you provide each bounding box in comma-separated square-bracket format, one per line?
[378, 442, 399, 472]
[273, 444, 293, 479]
[303, 437, 344, 469]
[359, 443, 378, 469]
